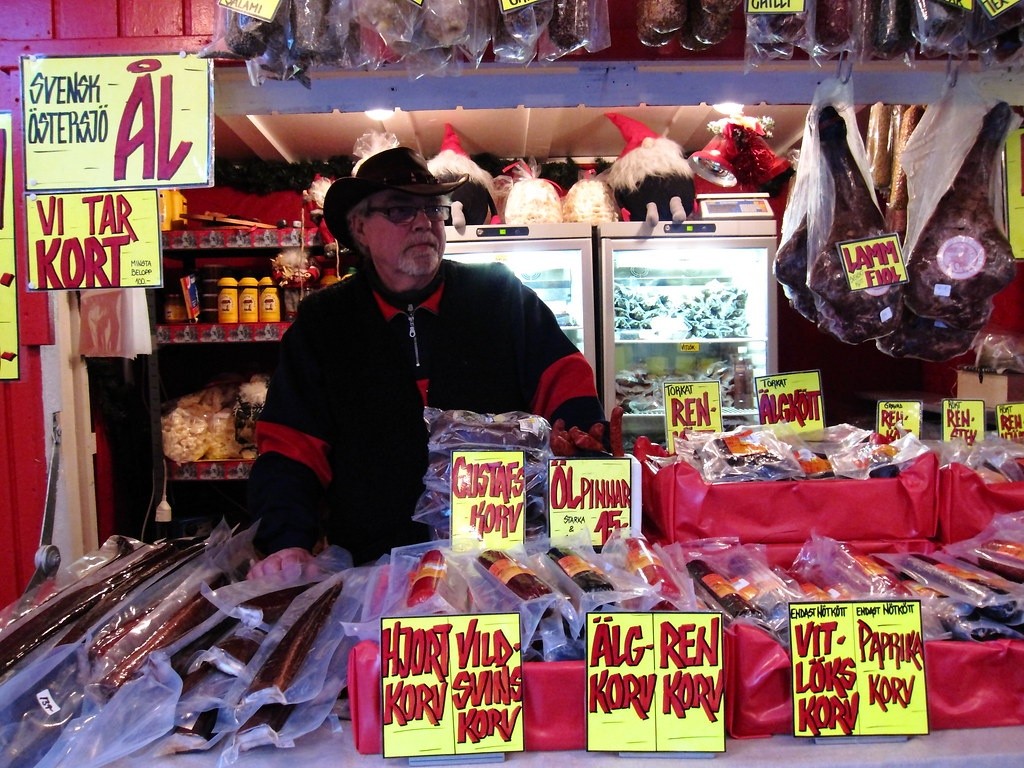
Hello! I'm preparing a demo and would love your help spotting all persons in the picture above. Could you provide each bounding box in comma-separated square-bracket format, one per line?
[240, 147, 614, 567]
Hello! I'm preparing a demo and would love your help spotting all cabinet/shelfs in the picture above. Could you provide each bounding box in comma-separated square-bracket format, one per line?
[150, 218, 339, 484]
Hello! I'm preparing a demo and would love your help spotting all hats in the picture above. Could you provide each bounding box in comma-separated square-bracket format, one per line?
[323, 147, 469, 251]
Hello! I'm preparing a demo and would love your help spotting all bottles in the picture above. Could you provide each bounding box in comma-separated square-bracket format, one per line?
[216, 274, 280, 323]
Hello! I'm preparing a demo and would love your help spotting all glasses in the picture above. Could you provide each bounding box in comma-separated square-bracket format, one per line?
[364, 205, 453, 226]
